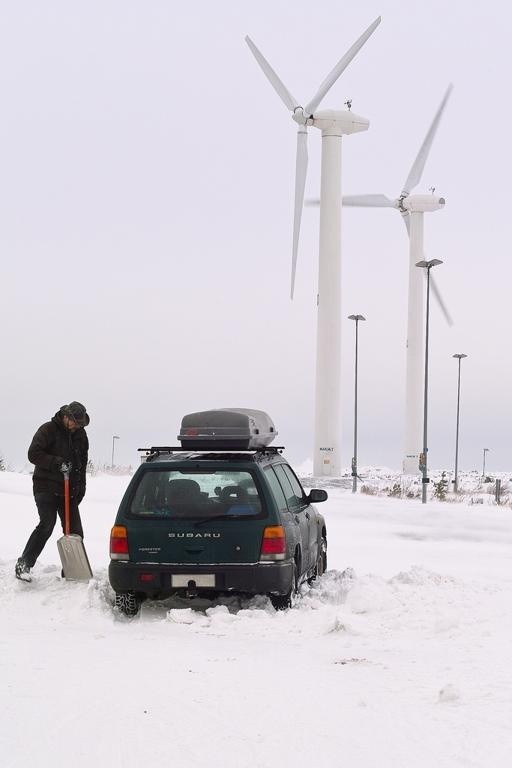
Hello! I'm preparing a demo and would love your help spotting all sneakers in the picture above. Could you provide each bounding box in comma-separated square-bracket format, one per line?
[15, 559, 31, 582]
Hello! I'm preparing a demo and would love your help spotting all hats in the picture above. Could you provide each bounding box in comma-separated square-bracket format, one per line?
[59, 401, 90, 428]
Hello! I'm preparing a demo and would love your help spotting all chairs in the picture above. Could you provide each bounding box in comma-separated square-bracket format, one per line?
[164, 478, 208, 519]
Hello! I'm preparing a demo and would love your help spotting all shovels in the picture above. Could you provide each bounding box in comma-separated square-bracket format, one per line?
[57, 472, 93, 583]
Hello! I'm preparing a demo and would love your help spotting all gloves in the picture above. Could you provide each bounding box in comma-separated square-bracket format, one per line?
[59, 459, 73, 473]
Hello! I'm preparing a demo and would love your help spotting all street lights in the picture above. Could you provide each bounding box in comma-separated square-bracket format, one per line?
[417, 259, 443, 503]
[483, 449, 488, 473]
[453, 353, 466, 493]
[347, 314, 366, 492]
[112, 436, 120, 468]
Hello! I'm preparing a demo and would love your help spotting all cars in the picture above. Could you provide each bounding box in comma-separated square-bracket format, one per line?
[108, 445, 328, 615]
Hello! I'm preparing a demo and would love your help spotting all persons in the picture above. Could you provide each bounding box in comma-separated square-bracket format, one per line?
[14, 400, 91, 582]
